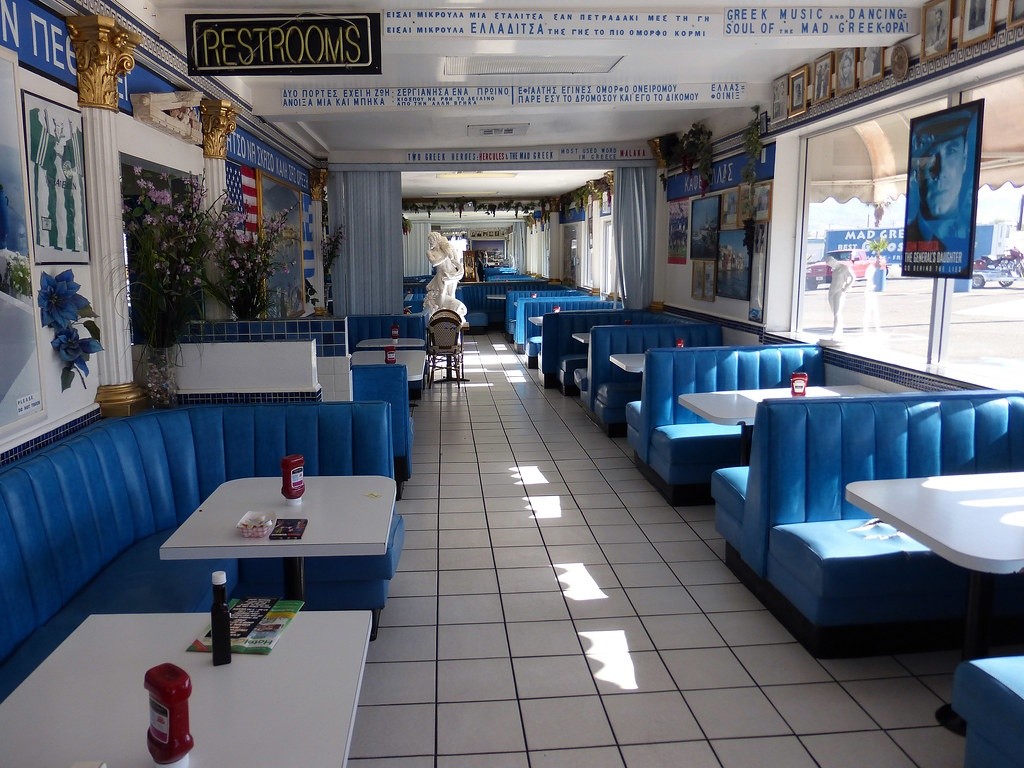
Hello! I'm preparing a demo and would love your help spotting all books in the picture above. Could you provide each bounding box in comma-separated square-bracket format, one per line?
[188, 597, 304, 655]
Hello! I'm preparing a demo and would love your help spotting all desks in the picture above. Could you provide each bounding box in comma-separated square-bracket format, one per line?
[0, 612, 375, 768]
[607, 354, 645, 375]
[845, 470, 1024, 735]
[351, 351, 426, 407]
[678, 383, 884, 467]
[528, 316, 543, 327]
[485, 295, 507, 300]
[158, 474, 396, 603]
[356, 339, 426, 348]
[572, 332, 591, 346]
[426, 321, 470, 382]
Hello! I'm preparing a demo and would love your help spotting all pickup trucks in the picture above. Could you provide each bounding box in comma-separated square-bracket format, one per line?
[806, 249, 886, 290]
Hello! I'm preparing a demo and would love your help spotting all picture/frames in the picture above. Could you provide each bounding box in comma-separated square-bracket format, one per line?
[901, 97, 988, 280]
[959, 0, 996, 48]
[788, 64, 808, 118]
[770, 75, 789, 125]
[690, 195, 721, 261]
[810, 51, 832, 105]
[759, 111, 767, 136]
[920, 0, 953, 66]
[715, 227, 754, 302]
[859, 48, 886, 87]
[20, 88, 93, 267]
[691, 258, 717, 302]
[753, 181, 772, 222]
[735, 181, 752, 228]
[1006, 0, 1024, 31]
[834, 49, 856, 97]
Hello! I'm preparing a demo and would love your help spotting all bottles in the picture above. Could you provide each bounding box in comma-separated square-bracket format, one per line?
[532, 294, 537, 298]
[675, 338, 684, 348]
[624, 320, 631, 325]
[391, 324, 399, 340]
[554, 306, 560, 312]
[404, 308, 410, 314]
[210, 570, 231, 666]
[384, 346, 396, 364]
[407, 288, 412, 294]
[144, 662, 195, 768]
[280, 454, 306, 505]
[790, 372, 808, 398]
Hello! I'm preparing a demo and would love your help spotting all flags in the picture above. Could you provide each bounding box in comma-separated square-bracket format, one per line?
[223, 157, 258, 238]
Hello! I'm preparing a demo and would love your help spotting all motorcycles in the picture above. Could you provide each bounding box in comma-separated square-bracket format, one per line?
[971, 246, 1024, 289]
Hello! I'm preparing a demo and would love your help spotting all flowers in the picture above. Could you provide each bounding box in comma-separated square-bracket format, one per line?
[201, 202, 304, 319]
[37, 269, 106, 392]
[319, 222, 344, 282]
[117, 164, 220, 347]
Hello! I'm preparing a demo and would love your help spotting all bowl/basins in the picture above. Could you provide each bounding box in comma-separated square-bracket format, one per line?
[238, 510, 275, 539]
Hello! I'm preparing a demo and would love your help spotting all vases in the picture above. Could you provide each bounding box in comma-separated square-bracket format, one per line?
[324, 282, 330, 307]
[144, 344, 177, 410]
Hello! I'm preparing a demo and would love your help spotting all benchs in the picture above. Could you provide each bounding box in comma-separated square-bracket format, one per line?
[0, 274, 434, 704]
[456, 280, 1024, 768]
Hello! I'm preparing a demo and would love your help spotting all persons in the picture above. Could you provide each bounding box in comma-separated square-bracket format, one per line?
[816, 62, 829, 98]
[426, 232, 465, 311]
[476, 249, 488, 283]
[931, 9, 946, 43]
[862, 251, 887, 340]
[863, 47, 880, 77]
[823, 256, 855, 340]
[838, 50, 852, 88]
[910, 105, 967, 275]
[795, 83, 801, 104]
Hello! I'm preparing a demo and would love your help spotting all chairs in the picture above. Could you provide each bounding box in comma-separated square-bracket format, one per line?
[428, 310, 461, 390]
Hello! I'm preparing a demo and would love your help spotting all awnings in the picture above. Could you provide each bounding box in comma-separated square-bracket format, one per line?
[808, 71, 1024, 203]
